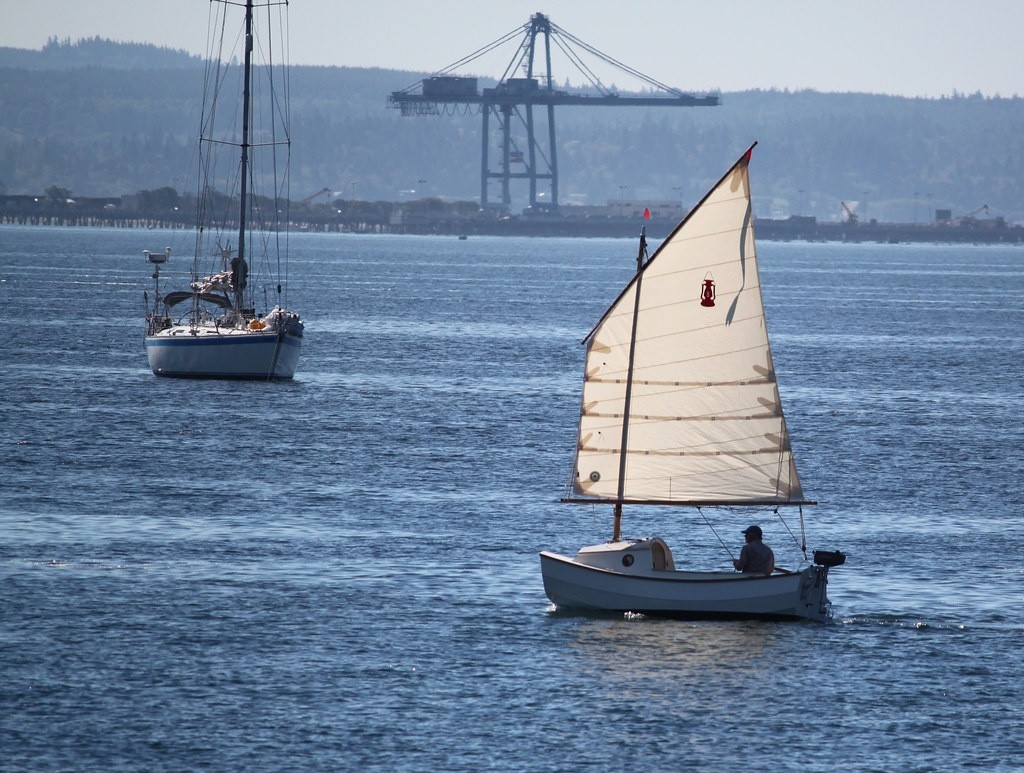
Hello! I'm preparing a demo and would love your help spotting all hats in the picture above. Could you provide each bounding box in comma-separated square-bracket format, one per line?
[741, 525, 762, 535]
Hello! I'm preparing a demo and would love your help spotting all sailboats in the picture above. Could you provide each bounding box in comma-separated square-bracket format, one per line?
[141, 1, 305, 384]
[537, 141, 848, 626]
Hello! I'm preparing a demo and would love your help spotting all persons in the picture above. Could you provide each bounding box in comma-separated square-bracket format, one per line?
[733, 526, 774, 573]
